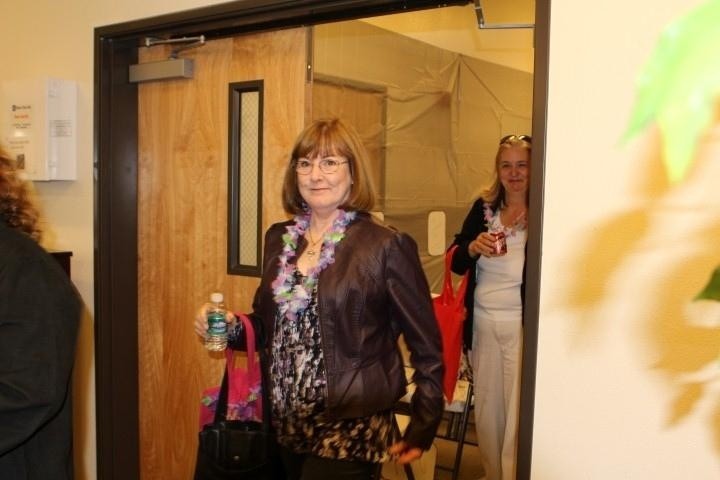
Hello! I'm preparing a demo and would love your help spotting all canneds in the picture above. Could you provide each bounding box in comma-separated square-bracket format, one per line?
[490, 231, 507, 257]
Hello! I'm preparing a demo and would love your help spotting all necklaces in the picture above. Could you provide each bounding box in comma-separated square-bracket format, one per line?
[480, 199, 529, 237]
[307, 222, 325, 255]
[272, 202, 358, 319]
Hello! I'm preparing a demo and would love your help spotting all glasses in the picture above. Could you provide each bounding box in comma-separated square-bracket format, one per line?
[499, 134, 532, 146]
[291, 157, 351, 175]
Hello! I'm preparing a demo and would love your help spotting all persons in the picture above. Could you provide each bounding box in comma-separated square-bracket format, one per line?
[0, 145, 83, 480]
[194, 115, 445, 479]
[446, 134, 532, 480]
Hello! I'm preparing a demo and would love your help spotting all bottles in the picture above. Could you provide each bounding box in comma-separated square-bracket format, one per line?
[205, 292, 228, 352]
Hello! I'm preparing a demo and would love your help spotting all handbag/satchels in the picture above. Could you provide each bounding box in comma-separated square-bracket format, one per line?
[430, 242, 473, 407]
[191, 311, 290, 480]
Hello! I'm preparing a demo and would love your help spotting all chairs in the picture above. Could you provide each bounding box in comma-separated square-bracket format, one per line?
[390, 347, 480, 478]
